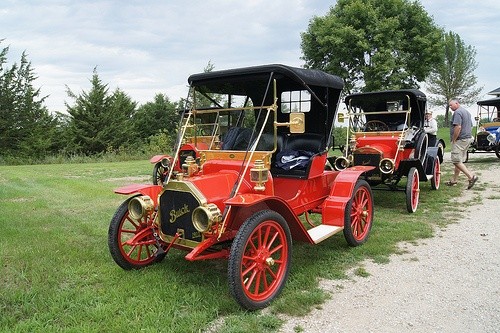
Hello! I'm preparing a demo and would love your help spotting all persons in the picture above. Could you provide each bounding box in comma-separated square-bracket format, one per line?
[444, 97, 478, 189]
[424, 111, 438, 148]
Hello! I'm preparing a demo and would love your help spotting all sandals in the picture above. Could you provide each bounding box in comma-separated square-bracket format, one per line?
[445, 180, 457, 186]
[468, 174, 478, 189]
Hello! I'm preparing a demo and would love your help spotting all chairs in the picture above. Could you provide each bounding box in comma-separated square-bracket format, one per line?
[237, 128, 325, 178]
